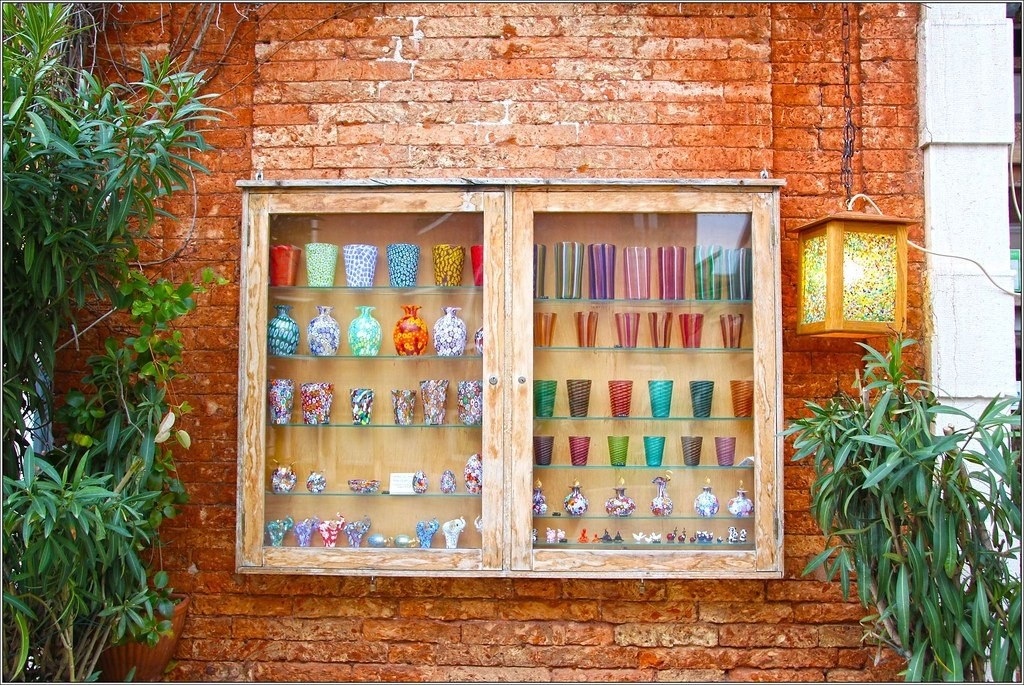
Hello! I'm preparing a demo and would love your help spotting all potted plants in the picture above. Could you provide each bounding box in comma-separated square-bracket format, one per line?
[49, 262, 231, 682]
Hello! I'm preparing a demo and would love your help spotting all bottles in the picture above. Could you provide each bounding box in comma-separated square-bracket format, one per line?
[269, 304, 482, 357]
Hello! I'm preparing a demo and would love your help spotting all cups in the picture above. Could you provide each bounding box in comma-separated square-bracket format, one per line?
[554, 241, 584, 298]
[533, 311, 754, 466]
[342, 245, 377, 287]
[267, 378, 482, 426]
[305, 243, 338, 286]
[658, 246, 687, 300]
[693, 246, 722, 299]
[386, 243, 419, 287]
[269, 245, 301, 286]
[433, 244, 466, 286]
[624, 247, 651, 300]
[588, 243, 615, 300]
[726, 247, 752, 301]
[471, 245, 484, 286]
[533, 243, 545, 298]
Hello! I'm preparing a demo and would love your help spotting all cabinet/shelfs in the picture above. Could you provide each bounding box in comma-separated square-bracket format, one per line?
[235, 178, 789, 578]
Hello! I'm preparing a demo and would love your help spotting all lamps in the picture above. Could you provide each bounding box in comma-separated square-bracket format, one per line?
[794, 3, 921, 338]
